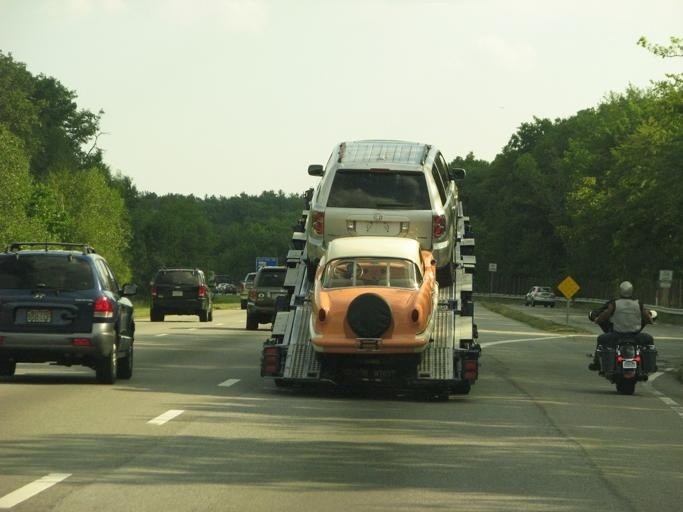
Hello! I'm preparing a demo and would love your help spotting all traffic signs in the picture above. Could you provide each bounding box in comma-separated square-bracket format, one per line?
[255, 256, 278, 272]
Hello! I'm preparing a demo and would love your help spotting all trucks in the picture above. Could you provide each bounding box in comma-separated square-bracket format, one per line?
[260, 189, 480, 404]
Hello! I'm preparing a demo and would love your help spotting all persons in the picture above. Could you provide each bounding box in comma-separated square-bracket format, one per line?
[587, 281, 657, 373]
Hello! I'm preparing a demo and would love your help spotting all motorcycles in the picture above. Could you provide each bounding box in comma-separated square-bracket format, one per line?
[585, 309, 657, 394]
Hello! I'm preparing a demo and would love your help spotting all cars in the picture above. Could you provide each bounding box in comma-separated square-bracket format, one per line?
[239, 273, 256, 309]
[215, 282, 237, 294]
[245, 266, 287, 330]
[524, 286, 555, 308]
[309, 235, 439, 364]
[302, 138, 466, 286]
[148, 267, 213, 322]
[0, 242, 134, 384]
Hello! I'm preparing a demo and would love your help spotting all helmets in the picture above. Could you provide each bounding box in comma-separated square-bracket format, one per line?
[619, 281, 633, 297]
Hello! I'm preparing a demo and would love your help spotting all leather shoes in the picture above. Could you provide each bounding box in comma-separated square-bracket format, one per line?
[589, 363, 601, 371]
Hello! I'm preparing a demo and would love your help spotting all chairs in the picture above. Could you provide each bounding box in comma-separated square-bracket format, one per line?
[324, 278, 364, 288]
[377, 278, 415, 288]
